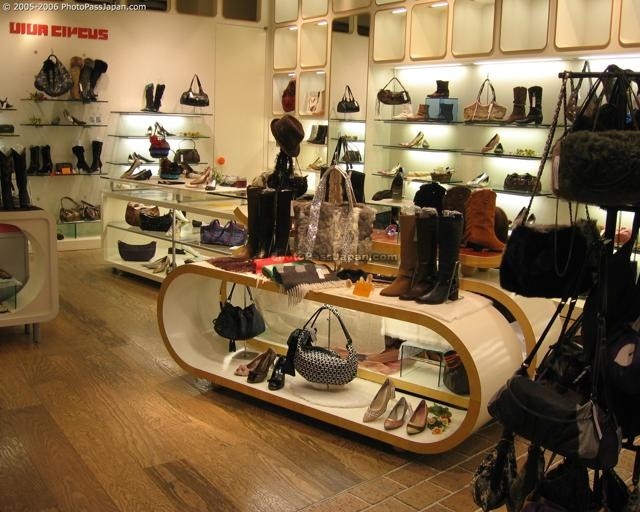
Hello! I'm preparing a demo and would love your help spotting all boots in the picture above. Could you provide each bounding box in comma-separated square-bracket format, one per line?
[392, 80, 453, 123]
[72, 146, 93, 173]
[413, 183, 506, 252]
[90, 141, 104, 173]
[0, 145, 31, 211]
[232, 185, 293, 262]
[27, 145, 53, 174]
[414, 213, 466, 304]
[307, 124, 328, 145]
[379, 210, 419, 298]
[70, 56, 108, 101]
[398, 209, 438, 303]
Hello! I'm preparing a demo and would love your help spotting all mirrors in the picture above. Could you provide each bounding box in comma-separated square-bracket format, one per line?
[325, 11, 372, 174]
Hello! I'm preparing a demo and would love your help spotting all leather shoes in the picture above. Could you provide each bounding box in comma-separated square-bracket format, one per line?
[372, 173, 403, 201]
[400, 131, 430, 148]
[363, 376, 428, 435]
[232, 346, 288, 391]
[0, 97, 14, 109]
[51, 116, 60, 124]
[63, 110, 87, 124]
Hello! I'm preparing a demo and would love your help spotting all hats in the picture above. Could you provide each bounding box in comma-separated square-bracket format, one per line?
[270, 114, 305, 157]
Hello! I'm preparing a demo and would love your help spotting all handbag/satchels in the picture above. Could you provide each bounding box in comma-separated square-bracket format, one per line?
[293, 164, 377, 263]
[117, 74, 247, 263]
[266, 150, 308, 200]
[213, 283, 266, 341]
[442, 347, 470, 396]
[341, 149, 362, 161]
[281, 79, 295, 111]
[337, 84, 360, 113]
[56, 163, 73, 172]
[470, 61, 640, 511]
[60, 196, 101, 221]
[33, 55, 73, 95]
[463, 77, 507, 123]
[319, 136, 366, 205]
[379, 76, 411, 105]
[0, 125, 14, 133]
[289, 304, 359, 385]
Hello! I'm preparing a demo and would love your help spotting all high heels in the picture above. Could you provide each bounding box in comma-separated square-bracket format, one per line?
[379, 162, 403, 175]
[142, 255, 178, 275]
[306, 156, 327, 170]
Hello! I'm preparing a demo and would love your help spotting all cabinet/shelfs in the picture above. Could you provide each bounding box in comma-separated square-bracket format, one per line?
[1, 91, 639, 457]
